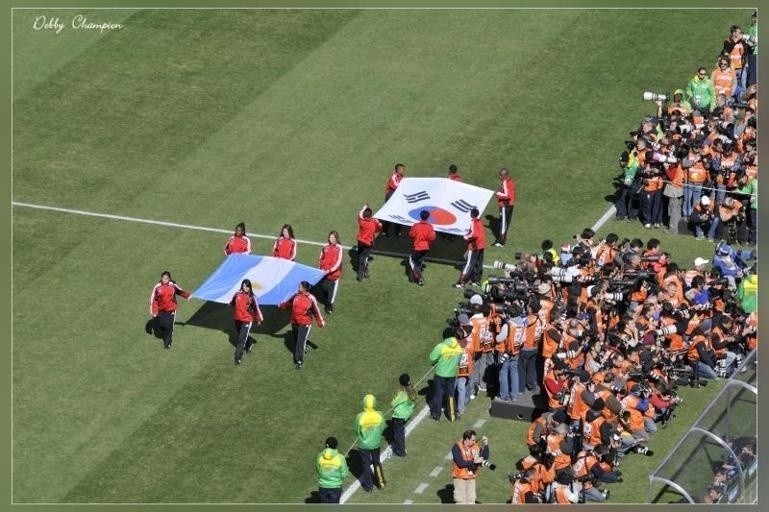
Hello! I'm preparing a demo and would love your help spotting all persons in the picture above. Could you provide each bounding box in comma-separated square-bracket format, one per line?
[229, 279, 264, 364]
[407, 210, 436, 285]
[353, 394, 387, 492]
[224, 221, 251, 256]
[380, 163, 406, 236]
[149, 270, 192, 349]
[273, 224, 297, 261]
[315, 437, 349, 503]
[390, 373, 417, 457]
[318, 230, 343, 314]
[279, 280, 325, 368]
[356, 204, 383, 280]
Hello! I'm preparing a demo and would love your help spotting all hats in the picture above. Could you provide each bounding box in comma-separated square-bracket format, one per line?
[701, 195, 710, 206]
[469, 294, 484, 306]
[692, 256, 709, 267]
[399, 373, 411, 386]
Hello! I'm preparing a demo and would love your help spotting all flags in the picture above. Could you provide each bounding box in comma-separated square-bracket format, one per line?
[372, 176, 495, 237]
[192, 254, 326, 307]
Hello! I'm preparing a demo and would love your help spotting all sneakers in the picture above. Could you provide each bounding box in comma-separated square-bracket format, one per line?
[455, 281, 465, 288]
[468, 281, 481, 288]
[325, 303, 332, 313]
[248, 343, 254, 352]
[305, 344, 309, 353]
[493, 240, 505, 248]
[295, 360, 303, 369]
[612, 214, 756, 247]
[167, 342, 175, 351]
[235, 357, 242, 364]
[430, 382, 537, 421]
[418, 278, 424, 285]
